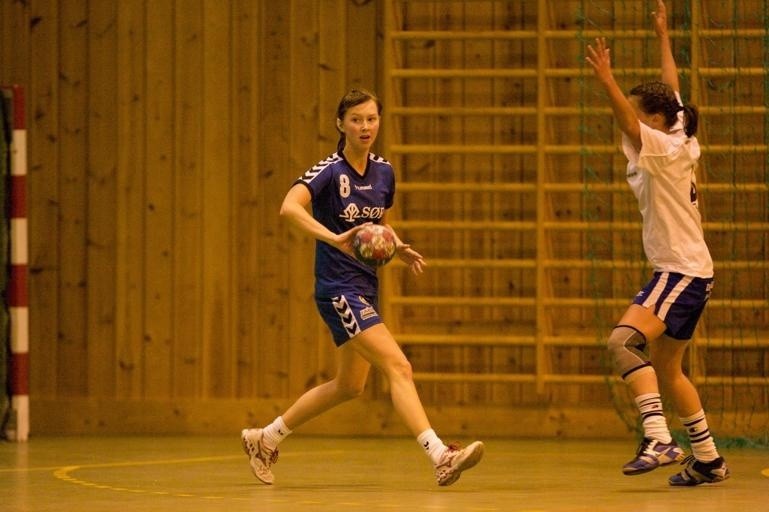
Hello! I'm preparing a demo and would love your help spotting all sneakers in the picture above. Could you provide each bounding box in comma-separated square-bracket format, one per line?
[435, 441, 485, 486]
[240, 427, 281, 485]
[667, 458, 730, 488]
[622, 436, 685, 475]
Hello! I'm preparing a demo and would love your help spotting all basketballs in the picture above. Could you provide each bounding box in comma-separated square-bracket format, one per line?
[354, 225, 397, 267]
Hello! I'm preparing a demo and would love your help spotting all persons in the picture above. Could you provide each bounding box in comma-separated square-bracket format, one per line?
[582, 2, 734, 488]
[240, 87, 484, 485]
